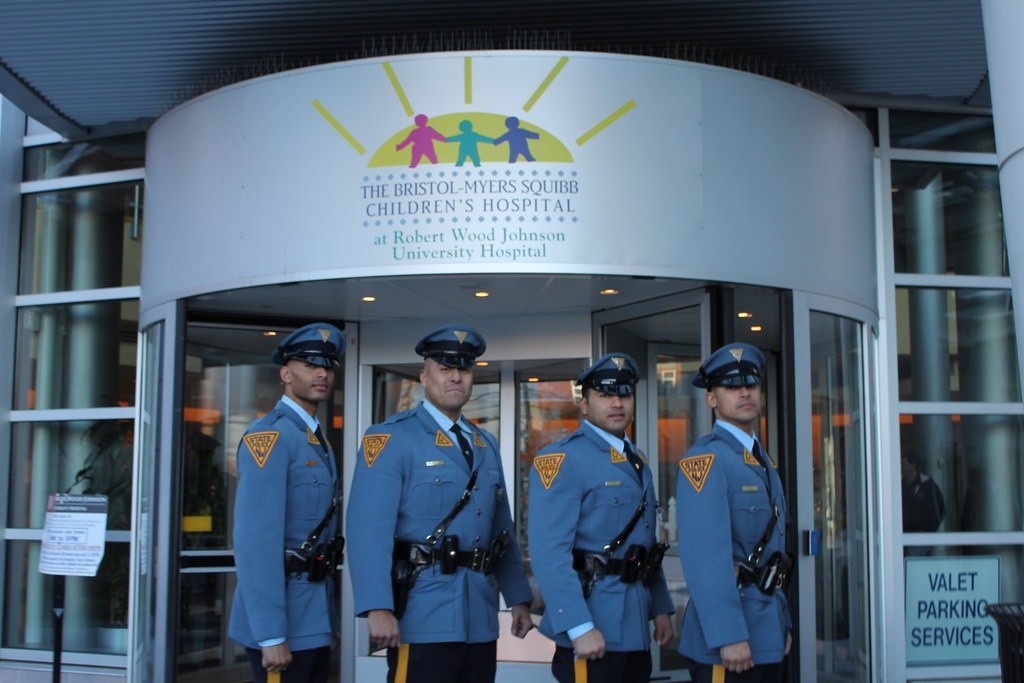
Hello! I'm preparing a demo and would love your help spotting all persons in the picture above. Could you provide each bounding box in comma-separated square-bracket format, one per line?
[226, 323, 346, 683]
[900, 451, 946, 557]
[527, 352, 676, 683]
[677, 343, 792, 683]
[346, 323, 534, 683]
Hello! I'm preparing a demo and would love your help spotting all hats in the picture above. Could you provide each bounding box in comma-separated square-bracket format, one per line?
[575, 352, 640, 397]
[415, 325, 486, 368]
[272, 322, 346, 370]
[193, 433, 221, 454]
[691, 343, 767, 388]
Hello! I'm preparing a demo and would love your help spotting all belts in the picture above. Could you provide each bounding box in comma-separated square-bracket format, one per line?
[735, 562, 785, 590]
[572, 553, 661, 582]
[392, 539, 498, 576]
[282, 545, 339, 582]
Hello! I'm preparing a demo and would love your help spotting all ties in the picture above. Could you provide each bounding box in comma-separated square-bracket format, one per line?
[752, 439, 771, 487]
[314, 424, 329, 461]
[623, 440, 643, 487]
[449, 423, 473, 470]
